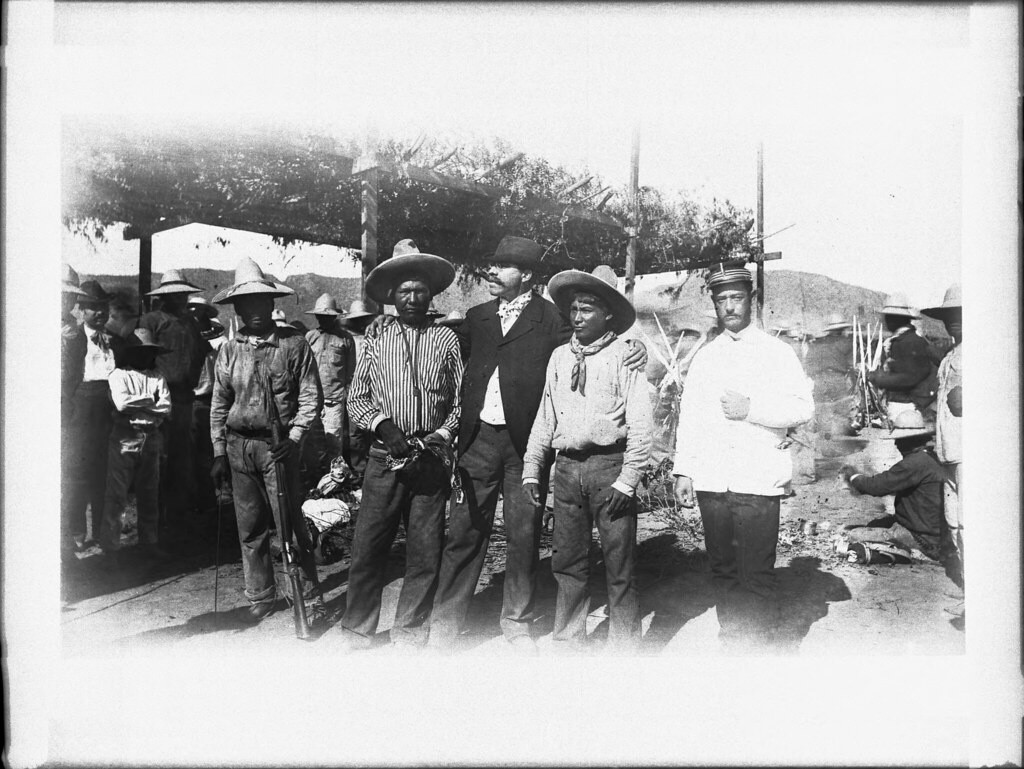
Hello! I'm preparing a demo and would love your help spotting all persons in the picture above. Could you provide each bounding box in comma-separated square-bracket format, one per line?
[62, 266, 226, 617]
[673, 263, 815, 644]
[522, 262, 654, 644]
[272, 294, 375, 562]
[210, 257, 322, 625]
[425, 236, 648, 646]
[919, 282, 964, 619]
[844, 410, 953, 562]
[340, 239, 465, 646]
[862, 292, 932, 421]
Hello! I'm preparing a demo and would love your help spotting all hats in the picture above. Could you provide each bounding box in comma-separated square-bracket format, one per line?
[705, 259, 752, 291]
[76, 279, 116, 303]
[879, 410, 936, 439]
[61, 263, 89, 297]
[920, 286, 963, 320]
[144, 268, 202, 295]
[122, 327, 162, 353]
[211, 258, 294, 304]
[481, 236, 552, 274]
[304, 292, 348, 315]
[188, 308, 225, 340]
[821, 312, 853, 330]
[877, 295, 923, 320]
[342, 300, 375, 319]
[187, 296, 218, 319]
[271, 309, 298, 331]
[437, 310, 466, 326]
[363, 239, 456, 306]
[546, 264, 636, 334]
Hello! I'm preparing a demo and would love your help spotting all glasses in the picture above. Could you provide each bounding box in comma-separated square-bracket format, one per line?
[487, 260, 509, 269]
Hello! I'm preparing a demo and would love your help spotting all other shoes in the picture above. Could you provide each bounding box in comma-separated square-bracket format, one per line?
[240, 594, 278, 623]
[513, 634, 540, 657]
[304, 601, 328, 627]
